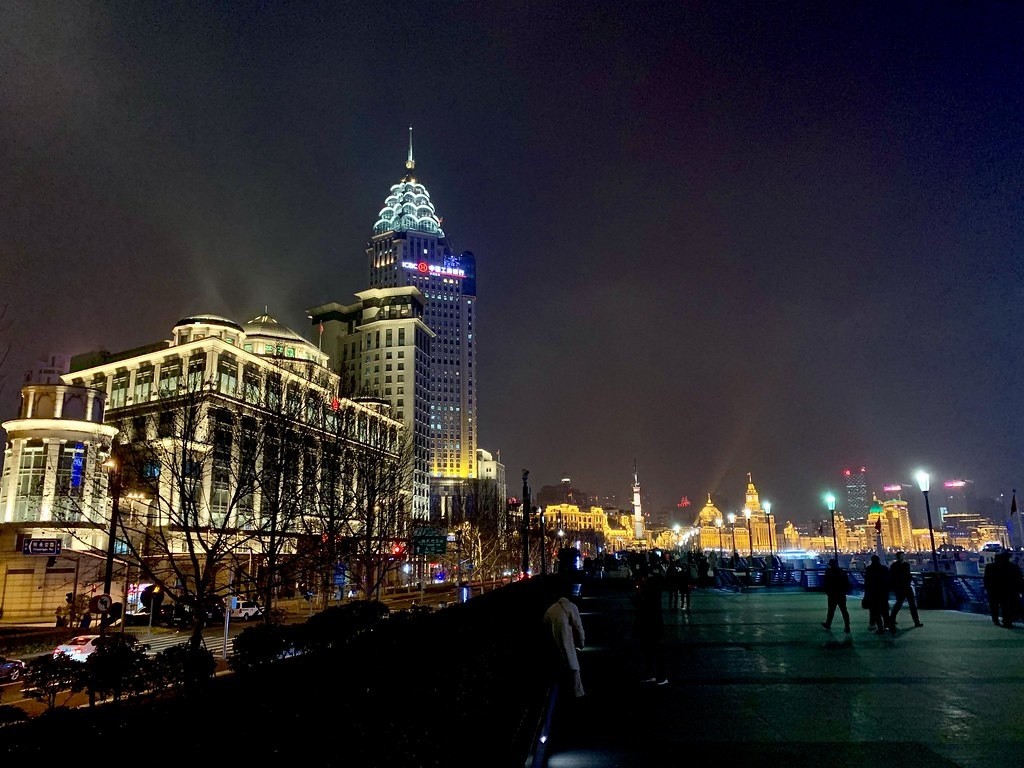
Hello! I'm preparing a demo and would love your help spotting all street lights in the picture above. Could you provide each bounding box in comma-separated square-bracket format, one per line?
[718, 519, 722, 557]
[764, 501, 772, 555]
[914, 469, 939, 572]
[729, 513, 736, 550]
[746, 509, 753, 556]
[674, 524, 700, 552]
[826, 492, 839, 569]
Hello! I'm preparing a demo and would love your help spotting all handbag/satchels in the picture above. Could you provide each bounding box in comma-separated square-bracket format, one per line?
[688, 584, 694, 591]
[837, 573, 849, 595]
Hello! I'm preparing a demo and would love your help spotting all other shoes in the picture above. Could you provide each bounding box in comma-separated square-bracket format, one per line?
[820, 622, 831, 630]
[576, 690, 584, 697]
[876, 629, 885, 634]
[868, 625, 876, 629]
[892, 628, 897, 636]
[915, 623, 923, 627]
[844, 628, 850, 633]
[893, 620, 898, 624]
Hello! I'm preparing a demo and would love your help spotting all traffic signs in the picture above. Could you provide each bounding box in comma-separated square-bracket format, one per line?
[414, 526, 448, 555]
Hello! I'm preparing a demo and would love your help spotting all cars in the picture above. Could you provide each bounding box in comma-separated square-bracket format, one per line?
[230, 599, 264, 618]
[0, 655, 27, 682]
[53, 634, 114, 661]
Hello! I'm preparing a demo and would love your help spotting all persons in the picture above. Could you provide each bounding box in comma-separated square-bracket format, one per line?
[820, 551, 924, 634]
[627, 546, 719, 610]
[733, 549, 739, 560]
[984, 549, 1024, 628]
[543, 584, 589, 703]
